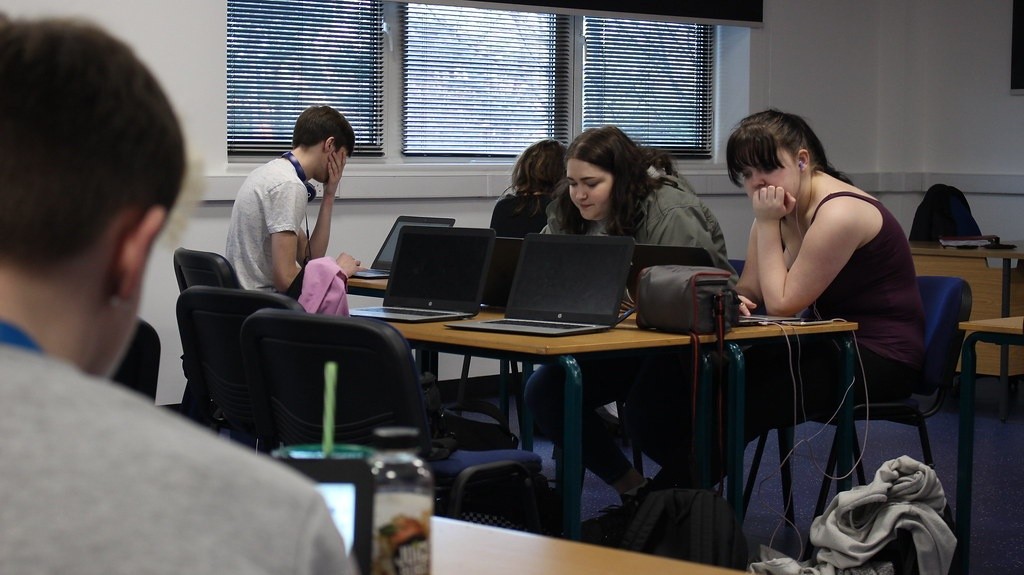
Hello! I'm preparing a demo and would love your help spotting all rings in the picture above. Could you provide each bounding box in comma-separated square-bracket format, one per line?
[356, 261, 361, 266]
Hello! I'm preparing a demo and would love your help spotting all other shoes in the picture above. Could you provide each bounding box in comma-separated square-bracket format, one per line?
[620, 478, 654, 505]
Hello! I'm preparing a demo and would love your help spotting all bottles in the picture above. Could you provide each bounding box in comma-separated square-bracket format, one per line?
[369, 429, 431, 575]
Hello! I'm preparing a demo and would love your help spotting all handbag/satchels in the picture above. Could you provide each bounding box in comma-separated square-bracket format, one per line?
[635, 264, 733, 334]
[419, 372, 518, 453]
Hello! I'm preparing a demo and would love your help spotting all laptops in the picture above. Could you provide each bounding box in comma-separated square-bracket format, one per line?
[277, 456, 372, 575]
[346, 216, 807, 337]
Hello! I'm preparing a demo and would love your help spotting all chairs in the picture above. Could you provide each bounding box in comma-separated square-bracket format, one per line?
[174, 246, 973, 556]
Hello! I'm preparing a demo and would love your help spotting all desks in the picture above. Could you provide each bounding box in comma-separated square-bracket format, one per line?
[951, 315, 1024, 575]
[719, 317, 859, 527]
[346, 276, 388, 298]
[430, 511, 748, 575]
[382, 313, 719, 544]
[905, 243, 1024, 420]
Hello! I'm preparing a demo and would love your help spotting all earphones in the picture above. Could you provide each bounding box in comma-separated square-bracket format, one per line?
[799, 160, 803, 169]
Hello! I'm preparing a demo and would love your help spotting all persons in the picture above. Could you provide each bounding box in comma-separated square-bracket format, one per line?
[491, 140, 568, 239]
[525, 127, 738, 518]
[576, 110, 924, 525]
[0, 13, 346, 575]
[225, 104, 368, 299]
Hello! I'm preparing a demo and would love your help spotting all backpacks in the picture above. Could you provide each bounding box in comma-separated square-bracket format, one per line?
[618, 487, 748, 571]
[285, 256, 349, 315]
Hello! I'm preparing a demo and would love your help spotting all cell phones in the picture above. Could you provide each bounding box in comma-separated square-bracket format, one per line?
[782, 320, 834, 326]
